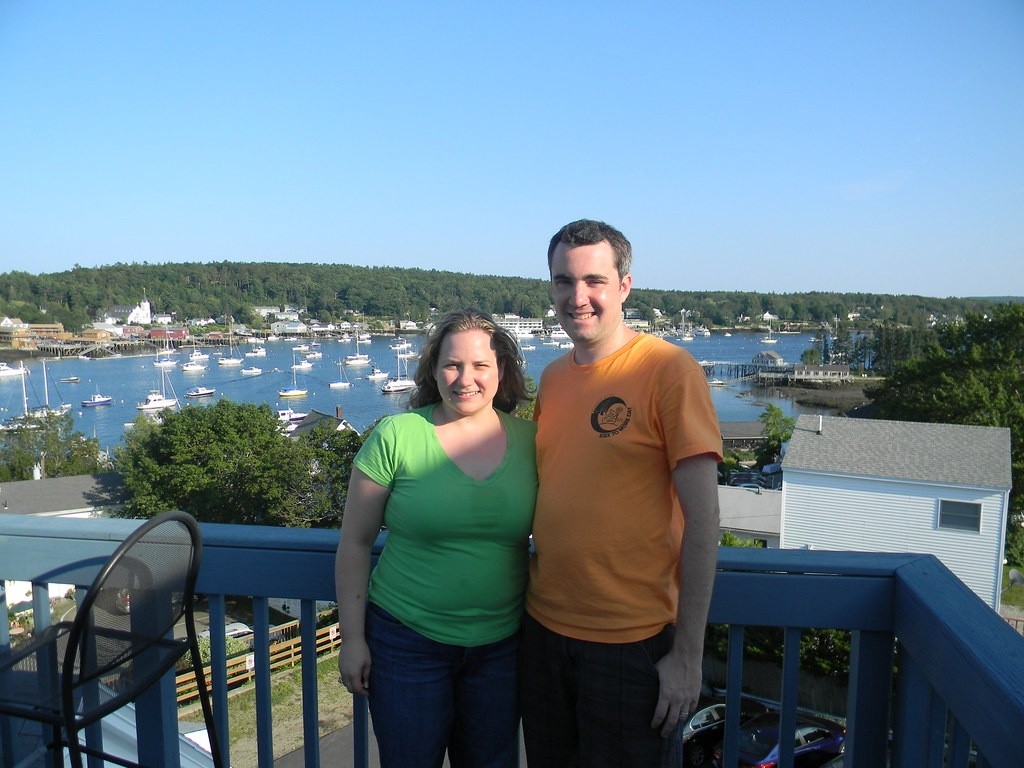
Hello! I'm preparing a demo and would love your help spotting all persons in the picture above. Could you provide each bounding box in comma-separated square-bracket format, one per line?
[335, 308, 539, 768]
[522, 221, 725, 768]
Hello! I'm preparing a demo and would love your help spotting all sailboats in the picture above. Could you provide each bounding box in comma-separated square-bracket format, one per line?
[628, 317, 842, 344]
[0, 321, 420, 464]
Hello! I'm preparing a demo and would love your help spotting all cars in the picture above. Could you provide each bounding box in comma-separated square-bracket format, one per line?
[115, 588, 200, 615]
[676, 696, 849, 768]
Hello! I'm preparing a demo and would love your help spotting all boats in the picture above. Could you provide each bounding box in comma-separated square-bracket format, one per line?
[513, 334, 577, 352]
[705, 377, 724, 387]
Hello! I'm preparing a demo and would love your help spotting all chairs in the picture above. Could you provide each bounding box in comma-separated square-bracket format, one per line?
[0, 510, 224, 768]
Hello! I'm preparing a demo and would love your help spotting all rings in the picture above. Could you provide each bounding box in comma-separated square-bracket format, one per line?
[680, 711, 689, 716]
[338, 677, 345, 683]
